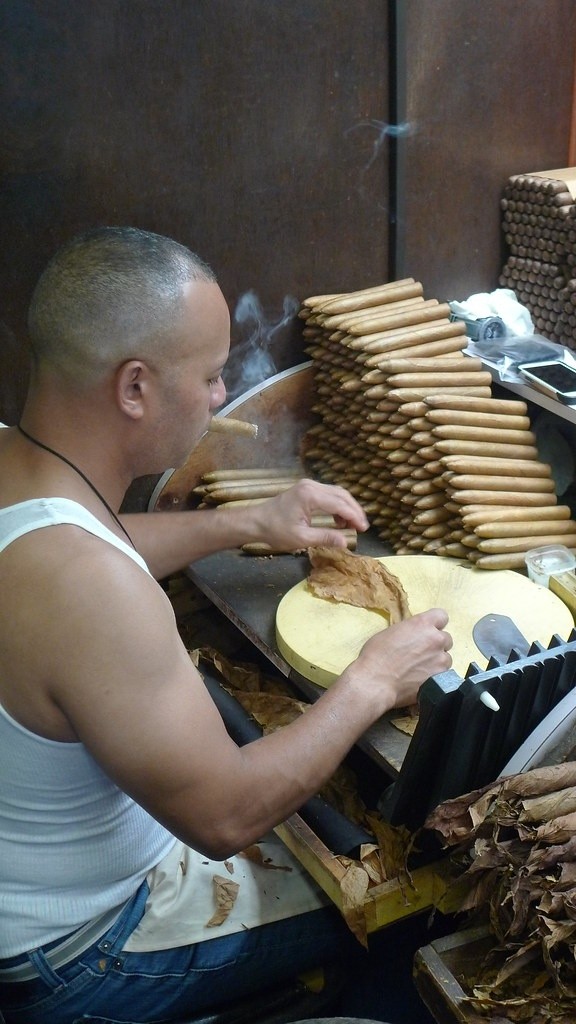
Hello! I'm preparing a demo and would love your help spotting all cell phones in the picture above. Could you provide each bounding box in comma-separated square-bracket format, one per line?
[516, 359, 576, 405]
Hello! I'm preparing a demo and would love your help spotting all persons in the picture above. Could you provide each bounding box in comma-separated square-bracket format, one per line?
[0, 224, 453, 1024]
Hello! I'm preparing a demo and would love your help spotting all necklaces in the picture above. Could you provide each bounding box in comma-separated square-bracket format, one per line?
[17, 415, 137, 552]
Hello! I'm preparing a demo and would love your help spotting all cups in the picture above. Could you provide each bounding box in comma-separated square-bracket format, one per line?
[524, 544, 576, 589]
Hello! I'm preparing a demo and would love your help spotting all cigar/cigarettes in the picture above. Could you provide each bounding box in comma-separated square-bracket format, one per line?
[192, 277, 576, 570]
[208, 416, 258, 440]
[496, 166, 576, 350]
